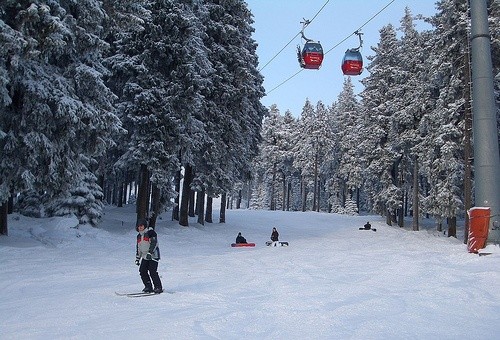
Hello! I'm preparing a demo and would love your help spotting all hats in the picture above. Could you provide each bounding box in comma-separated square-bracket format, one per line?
[135, 217, 148, 230]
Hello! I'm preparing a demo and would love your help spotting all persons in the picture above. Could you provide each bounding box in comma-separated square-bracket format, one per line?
[364, 221, 371, 230]
[134, 218, 163, 293]
[236, 232, 248, 244]
[270, 227, 279, 242]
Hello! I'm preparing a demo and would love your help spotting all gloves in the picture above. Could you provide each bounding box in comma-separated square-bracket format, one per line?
[135, 260, 140, 266]
[146, 254, 152, 259]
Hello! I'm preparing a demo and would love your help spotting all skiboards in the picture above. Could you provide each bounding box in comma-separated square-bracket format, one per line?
[115, 292, 159, 297]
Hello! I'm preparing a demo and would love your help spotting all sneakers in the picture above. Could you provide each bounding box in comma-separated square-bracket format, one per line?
[142, 284, 154, 293]
[152, 283, 164, 294]
